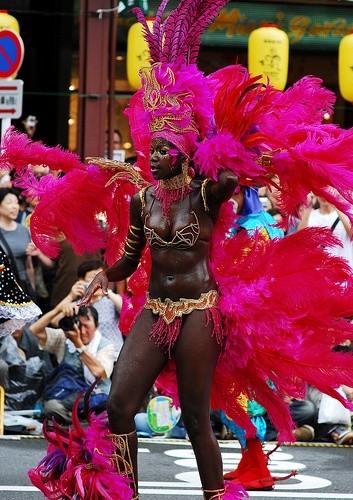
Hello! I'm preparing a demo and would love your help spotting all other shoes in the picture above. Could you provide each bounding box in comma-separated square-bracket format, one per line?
[292, 424, 315, 441]
[221, 424, 234, 440]
[328, 425, 353, 445]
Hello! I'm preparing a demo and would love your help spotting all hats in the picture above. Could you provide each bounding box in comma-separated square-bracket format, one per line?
[137, 62, 201, 161]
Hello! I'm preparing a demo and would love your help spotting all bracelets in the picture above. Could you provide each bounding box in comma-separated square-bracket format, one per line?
[107, 288, 112, 298]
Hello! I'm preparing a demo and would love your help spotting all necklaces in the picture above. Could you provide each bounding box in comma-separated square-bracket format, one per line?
[155, 170, 194, 222]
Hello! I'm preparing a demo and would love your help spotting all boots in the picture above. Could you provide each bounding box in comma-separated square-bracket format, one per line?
[229, 437, 275, 491]
[223, 448, 251, 480]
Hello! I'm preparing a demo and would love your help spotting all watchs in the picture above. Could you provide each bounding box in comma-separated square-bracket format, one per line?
[74, 344, 86, 357]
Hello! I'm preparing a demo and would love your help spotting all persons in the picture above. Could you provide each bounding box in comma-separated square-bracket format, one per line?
[219, 176, 286, 491]
[76, 122, 267, 500]
[1, 106, 353, 448]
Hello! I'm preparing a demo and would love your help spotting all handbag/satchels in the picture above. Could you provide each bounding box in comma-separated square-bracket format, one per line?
[41, 363, 94, 401]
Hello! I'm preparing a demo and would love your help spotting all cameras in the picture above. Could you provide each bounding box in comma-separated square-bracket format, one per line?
[59, 313, 82, 334]
